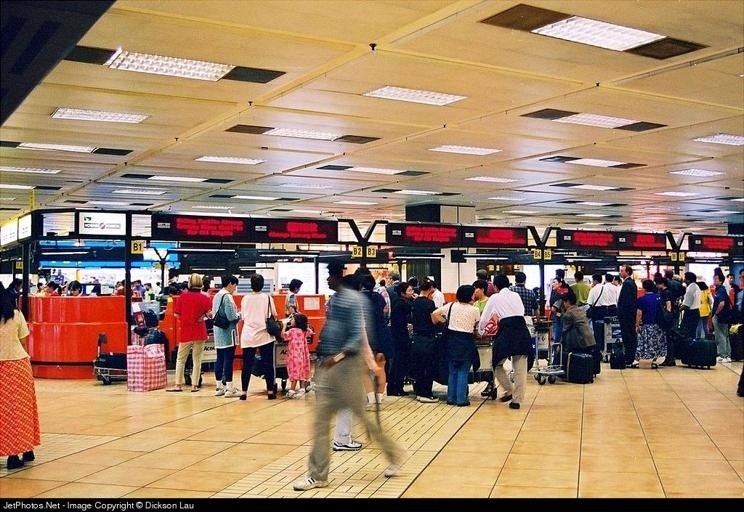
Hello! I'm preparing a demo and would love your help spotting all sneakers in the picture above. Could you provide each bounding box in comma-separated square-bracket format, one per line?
[625, 355, 732, 369]
[384, 468, 402, 479]
[332, 440, 364, 451]
[292, 476, 330, 490]
[166, 381, 306, 400]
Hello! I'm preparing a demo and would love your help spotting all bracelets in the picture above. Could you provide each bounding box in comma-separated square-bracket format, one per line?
[333, 352, 346, 363]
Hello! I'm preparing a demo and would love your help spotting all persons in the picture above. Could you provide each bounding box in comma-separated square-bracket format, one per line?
[7, 269, 84, 311]
[166, 273, 212, 392]
[617, 267, 684, 370]
[87, 276, 113, 293]
[0, 282, 41, 468]
[551, 265, 619, 364]
[115, 280, 163, 300]
[434, 269, 540, 406]
[211, 274, 241, 398]
[280, 279, 314, 401]
[681, 271, 744, 364]
[238, 275, 278, 396]
[292, 259, 438, 492]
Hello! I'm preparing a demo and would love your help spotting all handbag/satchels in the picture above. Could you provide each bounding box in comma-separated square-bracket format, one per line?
[213, 308, 230, 329]
[128, 344, 168, 392]
[718, 311, 741, 324]
[426, 322, 450, 344]
[267, 317, 282, 337]
[415, 336, 434, 352]
[611, 349, 625, 369]
[585, 306, 594, 317]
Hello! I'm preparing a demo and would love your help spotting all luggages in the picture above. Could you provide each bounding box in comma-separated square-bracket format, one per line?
[144, 328, 169, 370]
[554, 352, 594, 384]
[95, 352, 127, 369]
[728, 332, 744, 361]
[681, 338, 717, 368]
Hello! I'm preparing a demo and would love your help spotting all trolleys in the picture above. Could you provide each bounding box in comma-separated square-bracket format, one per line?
[531, 325, 564, 384]
[464, 370, 496, 400]
[91, 331, 127, 384]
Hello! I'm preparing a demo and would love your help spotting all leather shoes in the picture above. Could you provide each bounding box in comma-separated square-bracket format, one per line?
[7, 455, 23, 469]
[22, 451, 34, 462]
[366, 384, 520, 412]
